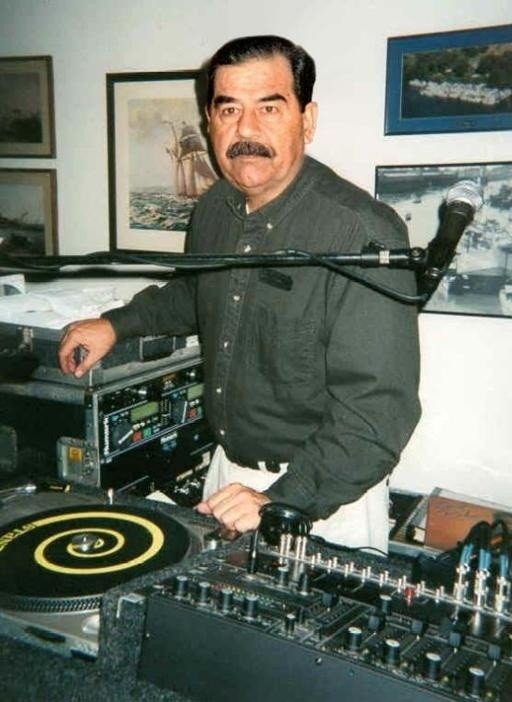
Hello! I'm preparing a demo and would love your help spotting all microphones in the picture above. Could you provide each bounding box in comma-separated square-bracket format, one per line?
[426, 180, 485, 287]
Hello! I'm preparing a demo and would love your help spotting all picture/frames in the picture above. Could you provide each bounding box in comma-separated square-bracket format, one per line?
[0, 56, 58, 158]
[385, 22, 512, 136]
[1, 169, 58, 263]
[106, 69, 223, 252]
[374, 161, 512, 318]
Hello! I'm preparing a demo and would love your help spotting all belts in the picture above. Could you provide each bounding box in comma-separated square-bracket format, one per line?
[218, 448, 293, 476]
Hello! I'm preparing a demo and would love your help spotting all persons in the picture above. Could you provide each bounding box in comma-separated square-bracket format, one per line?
[59, 35, 424, 560]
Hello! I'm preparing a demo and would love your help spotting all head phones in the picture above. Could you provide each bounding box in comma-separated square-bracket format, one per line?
[259, 499, 312, 546]
